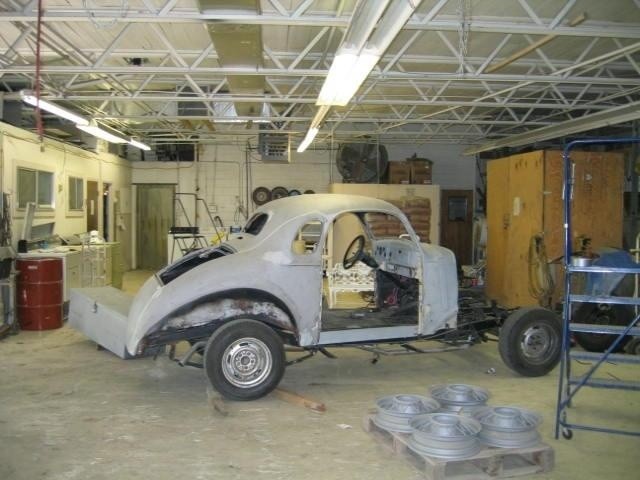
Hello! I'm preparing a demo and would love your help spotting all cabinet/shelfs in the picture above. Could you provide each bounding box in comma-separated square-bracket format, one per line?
[62, 243, 123, 320]
[298, 221, 330, 276]
[486, 149, 624, 312]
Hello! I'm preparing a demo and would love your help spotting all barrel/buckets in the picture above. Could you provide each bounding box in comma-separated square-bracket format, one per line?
[14, 260, 64, 330]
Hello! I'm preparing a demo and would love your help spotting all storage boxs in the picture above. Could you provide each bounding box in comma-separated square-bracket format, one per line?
[381, 160, 410, 184]
[406, 157, 433, 184]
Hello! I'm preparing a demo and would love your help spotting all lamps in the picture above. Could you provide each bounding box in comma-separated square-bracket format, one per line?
[313, 48, 380, 107]
[127, 137, 153, 153]
[294, 126, 321, 152]
[75, 118, 130, 147]
[21, 90, 88, 126]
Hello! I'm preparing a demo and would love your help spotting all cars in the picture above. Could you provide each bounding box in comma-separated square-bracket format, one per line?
[124, 194, 563, 400]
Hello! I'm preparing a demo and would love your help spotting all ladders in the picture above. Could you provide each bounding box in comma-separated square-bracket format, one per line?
[555, 137, 640, 440]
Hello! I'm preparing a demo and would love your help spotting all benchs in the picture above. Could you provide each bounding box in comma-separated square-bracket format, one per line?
[326, 263, 376, 308]
